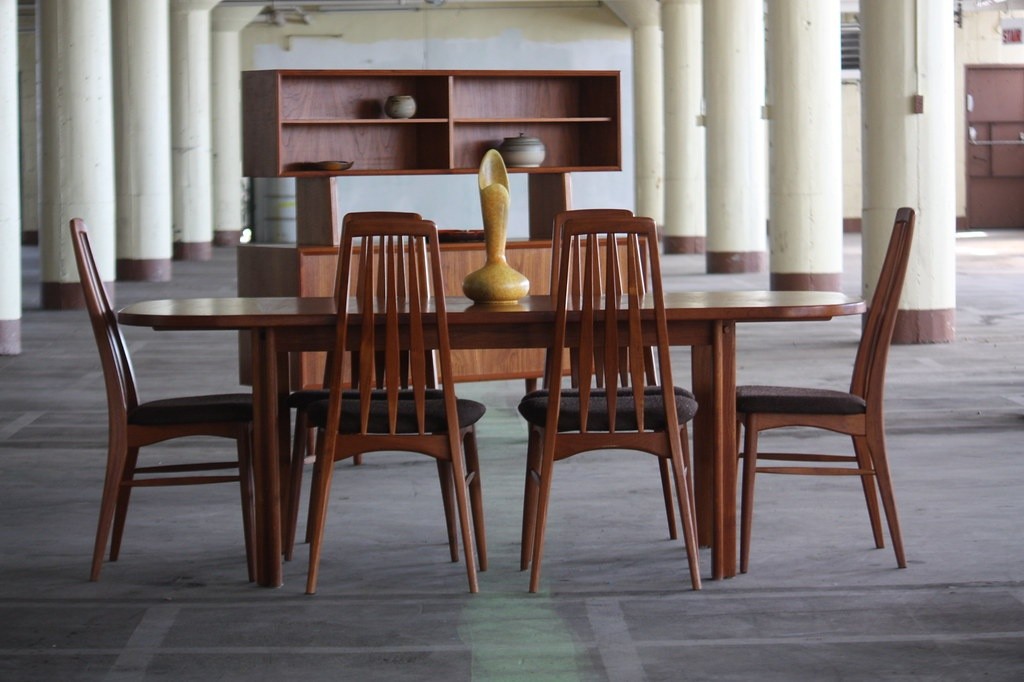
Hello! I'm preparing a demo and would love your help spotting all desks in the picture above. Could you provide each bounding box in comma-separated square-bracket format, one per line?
[117, 290, 867, 590]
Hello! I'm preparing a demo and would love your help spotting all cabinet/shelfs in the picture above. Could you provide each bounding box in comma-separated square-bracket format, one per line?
[242, 69, 624, 179]
[236, 237, 649, 465]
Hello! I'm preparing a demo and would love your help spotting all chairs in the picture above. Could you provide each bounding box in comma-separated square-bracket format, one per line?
[283, 209, 701, 595]
[736, 208, 915, 575]
[69, 218, 260, 583]
